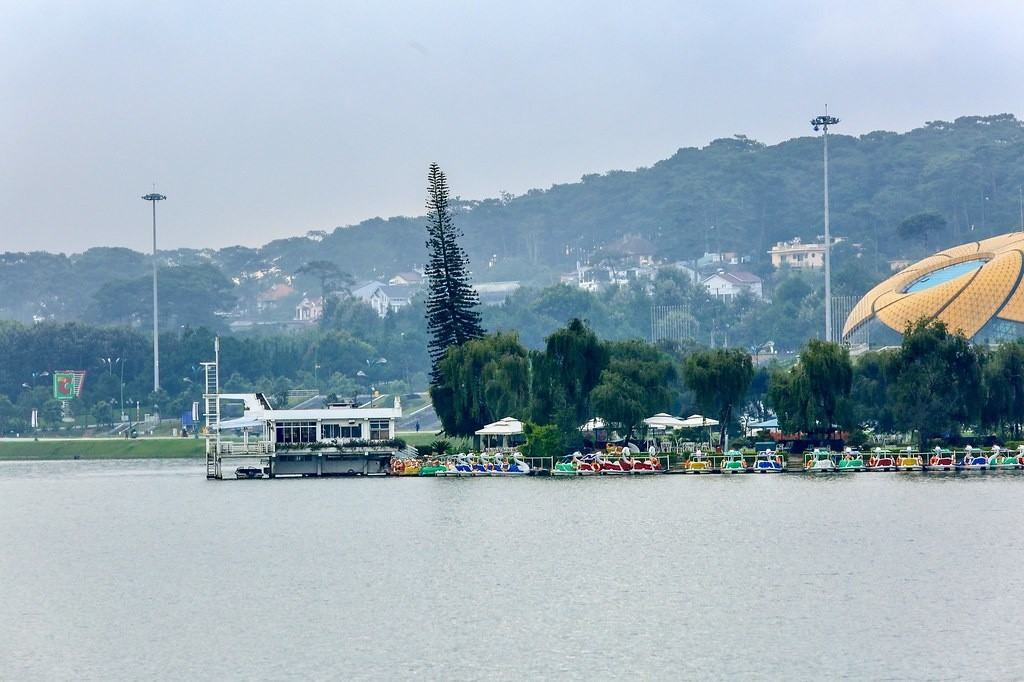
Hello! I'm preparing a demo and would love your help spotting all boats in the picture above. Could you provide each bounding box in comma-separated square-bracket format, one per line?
[802, 448, 837, 472]
[1012, 444, 1024, 469]
[835, 445, 866, 472]
[987, 443, 1022, 470]
[684, 449, 712, 474]
[389, 445, 667, 477]
[896, 446, 924, 471]
[956, 444, 990, 470]
[865, 447, 896, 472]
[720, 449, 748, 474]
[752, 448, 785, 472]
[924, 444, 957, 471]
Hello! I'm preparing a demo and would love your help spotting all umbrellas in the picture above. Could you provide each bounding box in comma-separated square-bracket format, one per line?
[638, 413, 720, 436]
[576, 417, 621, 441]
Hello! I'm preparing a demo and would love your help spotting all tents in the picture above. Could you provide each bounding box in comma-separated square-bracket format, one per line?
[474, 415, 534, 455]
[747, 413, 843, 441]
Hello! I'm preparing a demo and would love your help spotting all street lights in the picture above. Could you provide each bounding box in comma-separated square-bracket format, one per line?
[102, 357, 121, 376]
[811, 105, 842, 343]
[141, 180, 168, 392]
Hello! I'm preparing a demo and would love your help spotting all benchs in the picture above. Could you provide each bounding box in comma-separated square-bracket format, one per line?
[555, 462, 661, 470]
[689, 462, 782, 468]
[807, 457, 1019, 466]
[404, 464, 524, 472]
